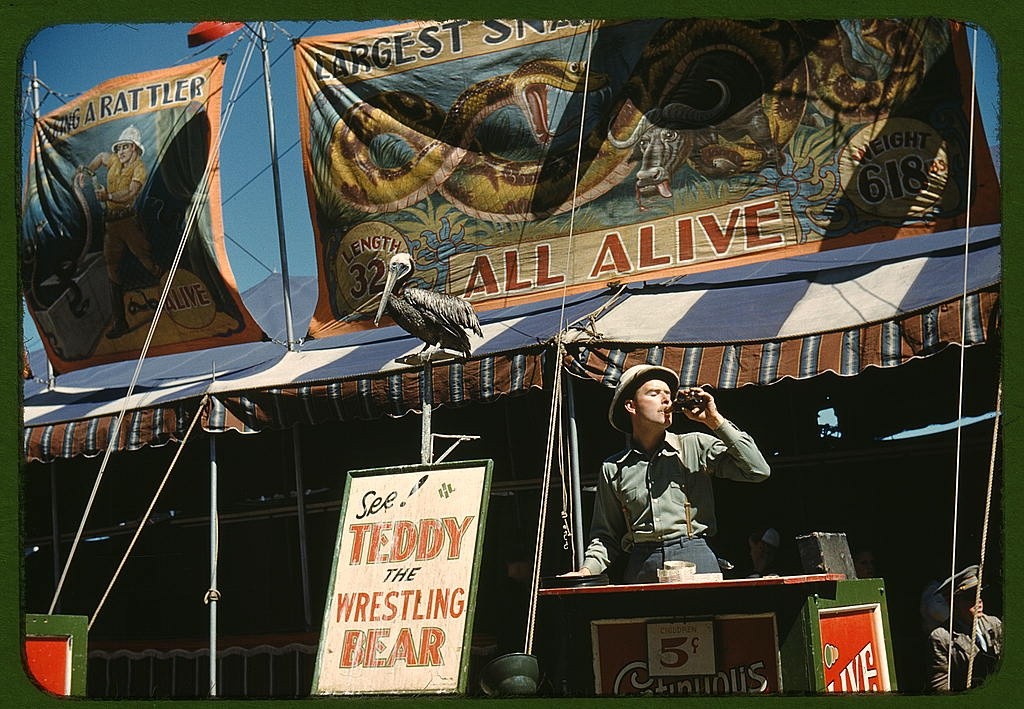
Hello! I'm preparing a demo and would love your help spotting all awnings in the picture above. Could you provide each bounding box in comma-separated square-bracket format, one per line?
[22, 223, 1003, 463]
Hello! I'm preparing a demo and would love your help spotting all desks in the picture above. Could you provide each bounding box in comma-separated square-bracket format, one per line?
[539, 577, 899, 696]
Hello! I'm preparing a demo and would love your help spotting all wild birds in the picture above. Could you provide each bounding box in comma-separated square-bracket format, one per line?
[373, 251, 482, 358]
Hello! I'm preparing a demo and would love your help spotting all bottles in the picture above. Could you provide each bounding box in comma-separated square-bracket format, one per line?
[664, 390, 707, 413]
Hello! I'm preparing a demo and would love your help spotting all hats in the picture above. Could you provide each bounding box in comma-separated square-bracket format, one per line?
[607, 363, 681, 434]
[932, 566, 991, 597]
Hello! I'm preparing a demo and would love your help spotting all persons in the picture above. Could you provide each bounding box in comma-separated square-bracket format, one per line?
[554, 364, 770, 584]
[490, 526, 539, 654]
[850, 544, 880, 580]
[919, 565, 1003, 695]
